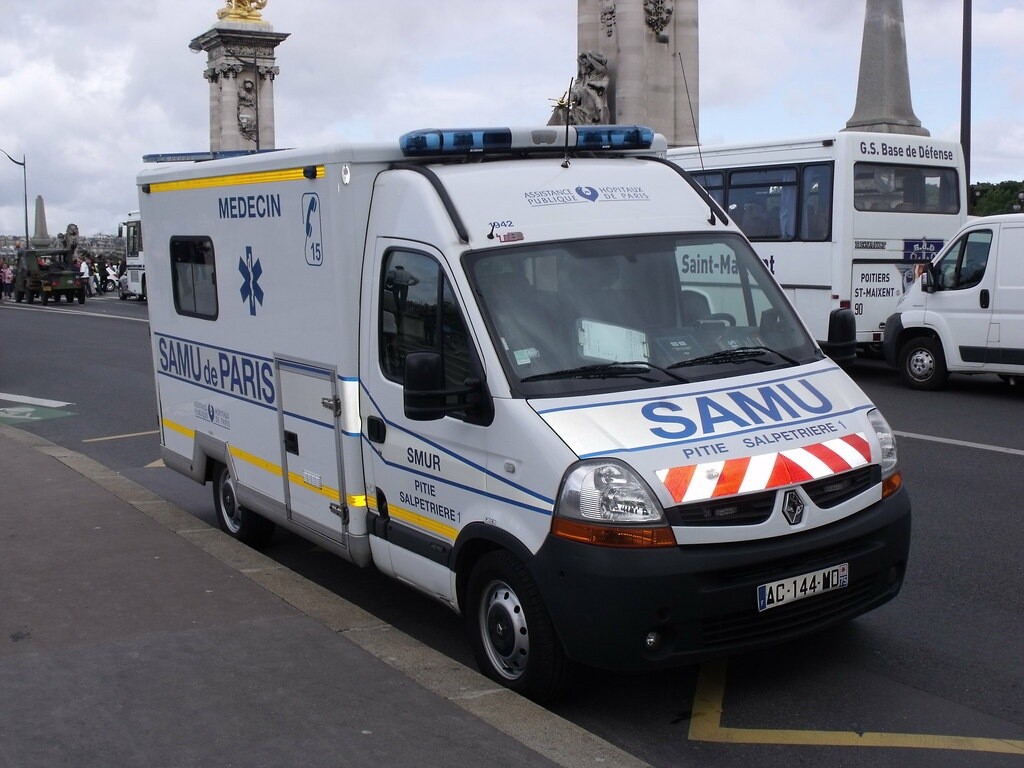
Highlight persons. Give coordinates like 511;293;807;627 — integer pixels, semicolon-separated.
382;264;437;376
0;256;19;303
73;250;127;299
559;51;612;127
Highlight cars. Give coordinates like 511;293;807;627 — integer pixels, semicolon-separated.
118;270;135;301
93;263;119;292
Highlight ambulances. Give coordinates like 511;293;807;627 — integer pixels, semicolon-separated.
136;53;913;691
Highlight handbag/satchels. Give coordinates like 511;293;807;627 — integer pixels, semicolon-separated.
99;270;110;277
385;271;397;291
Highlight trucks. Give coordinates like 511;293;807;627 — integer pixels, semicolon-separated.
883;213;1023;391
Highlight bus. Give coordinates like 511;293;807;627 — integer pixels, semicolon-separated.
668;132;967;357
118;210;147;301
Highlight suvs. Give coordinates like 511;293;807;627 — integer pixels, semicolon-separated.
14;248;85;305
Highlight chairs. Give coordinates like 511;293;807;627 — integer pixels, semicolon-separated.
487;272;546;343
571;259;647;317
735;201;831;239
855;200;958;215
